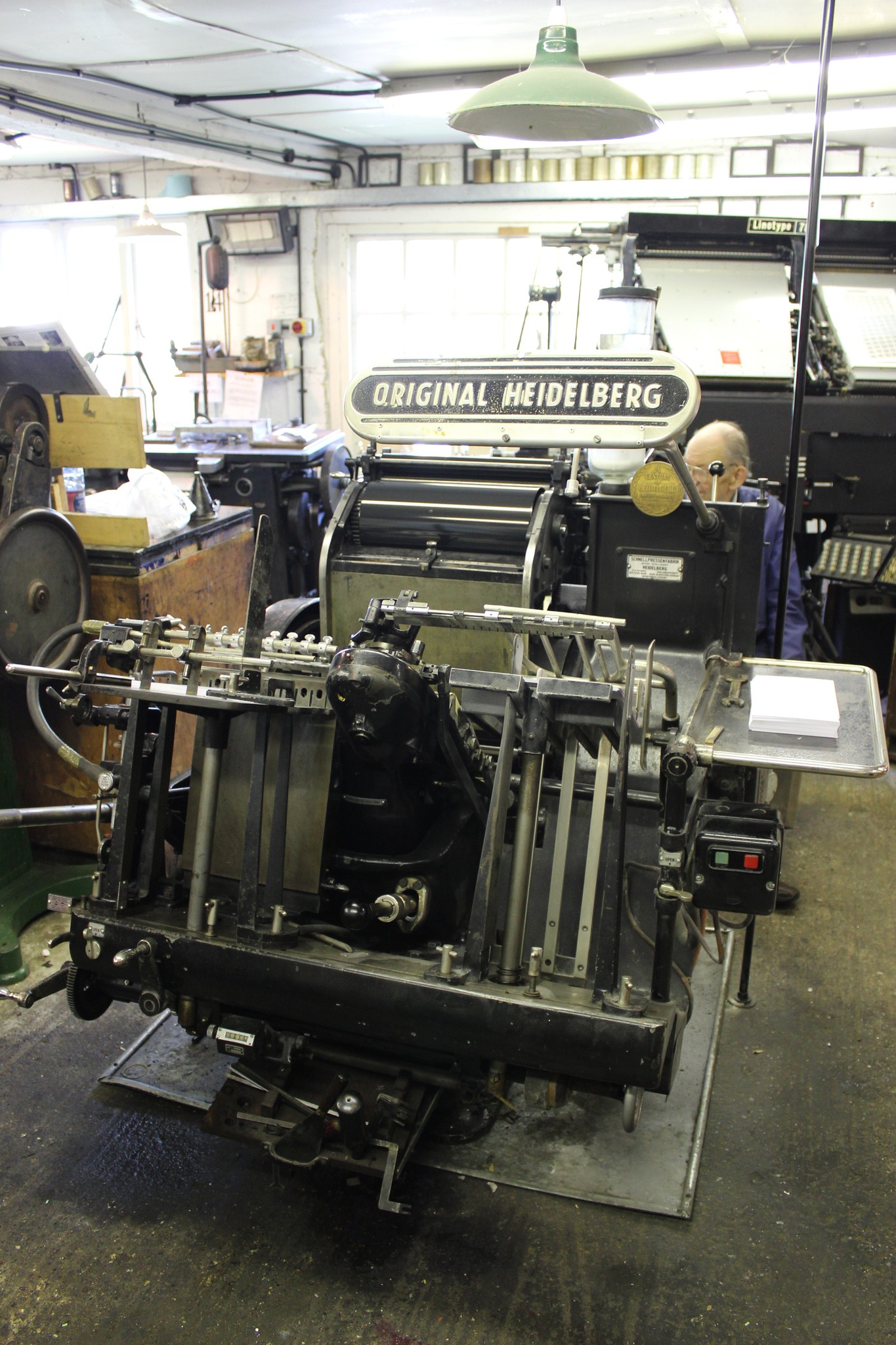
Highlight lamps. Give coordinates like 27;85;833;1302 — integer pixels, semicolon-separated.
119;156;181;239
445;1;665;143
206;206;295;257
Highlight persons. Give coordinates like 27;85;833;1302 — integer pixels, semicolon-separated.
683;421;808;661
